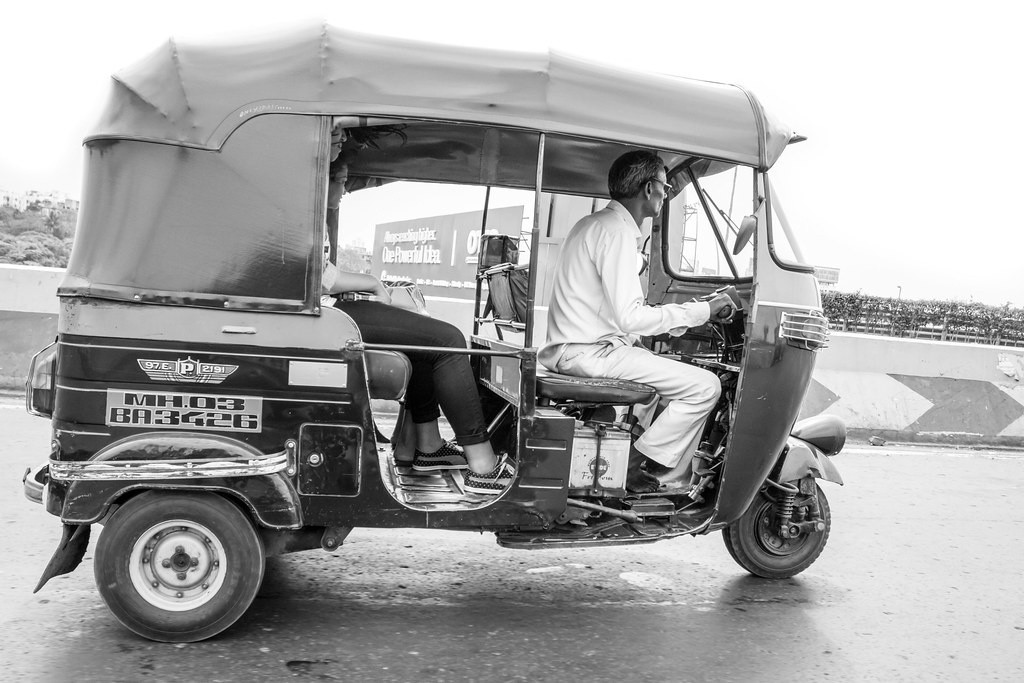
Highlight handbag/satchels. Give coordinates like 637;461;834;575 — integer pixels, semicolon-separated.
339;278;427;313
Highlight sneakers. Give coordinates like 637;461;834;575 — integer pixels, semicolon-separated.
413;437;469;471
464;453;518;495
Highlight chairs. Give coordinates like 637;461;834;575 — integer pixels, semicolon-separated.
485;262;654;411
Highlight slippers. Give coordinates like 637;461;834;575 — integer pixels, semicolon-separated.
394;459;413;467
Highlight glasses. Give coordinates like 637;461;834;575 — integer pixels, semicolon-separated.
641;176;674;194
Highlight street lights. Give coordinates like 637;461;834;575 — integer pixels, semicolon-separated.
897;285;902;298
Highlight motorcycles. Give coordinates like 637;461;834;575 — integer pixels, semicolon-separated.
21;21;853;646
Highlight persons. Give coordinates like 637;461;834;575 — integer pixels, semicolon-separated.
537;148;738;494
322;124;516;495
